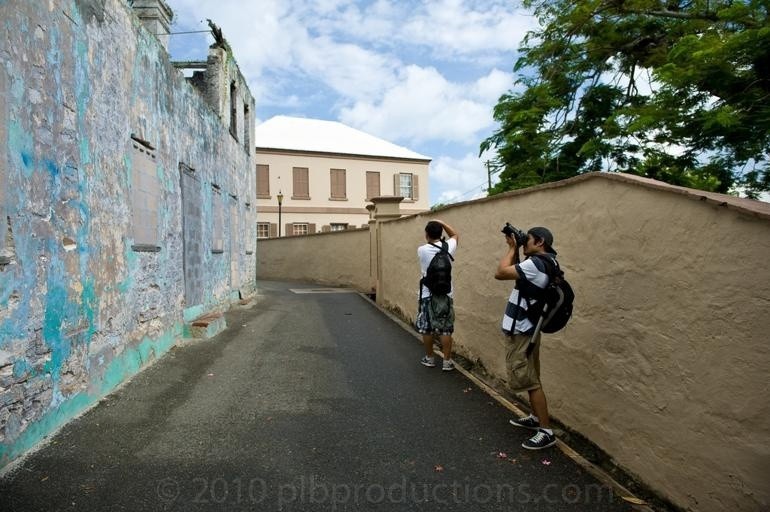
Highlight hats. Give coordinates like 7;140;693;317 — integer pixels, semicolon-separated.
528;226;557;255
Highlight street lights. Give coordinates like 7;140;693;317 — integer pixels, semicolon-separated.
277;190;284;237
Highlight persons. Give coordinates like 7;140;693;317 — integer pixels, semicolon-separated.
494;226;560;452
417;218;459;371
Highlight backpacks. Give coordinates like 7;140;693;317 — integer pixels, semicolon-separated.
419;240;451;312
527;255;575;334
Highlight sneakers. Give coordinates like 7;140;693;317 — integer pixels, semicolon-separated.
521;428;556;449
420;355;436;367
510;416;539;430
441;358;455;371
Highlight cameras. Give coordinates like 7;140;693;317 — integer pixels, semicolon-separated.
501;222;529;248
